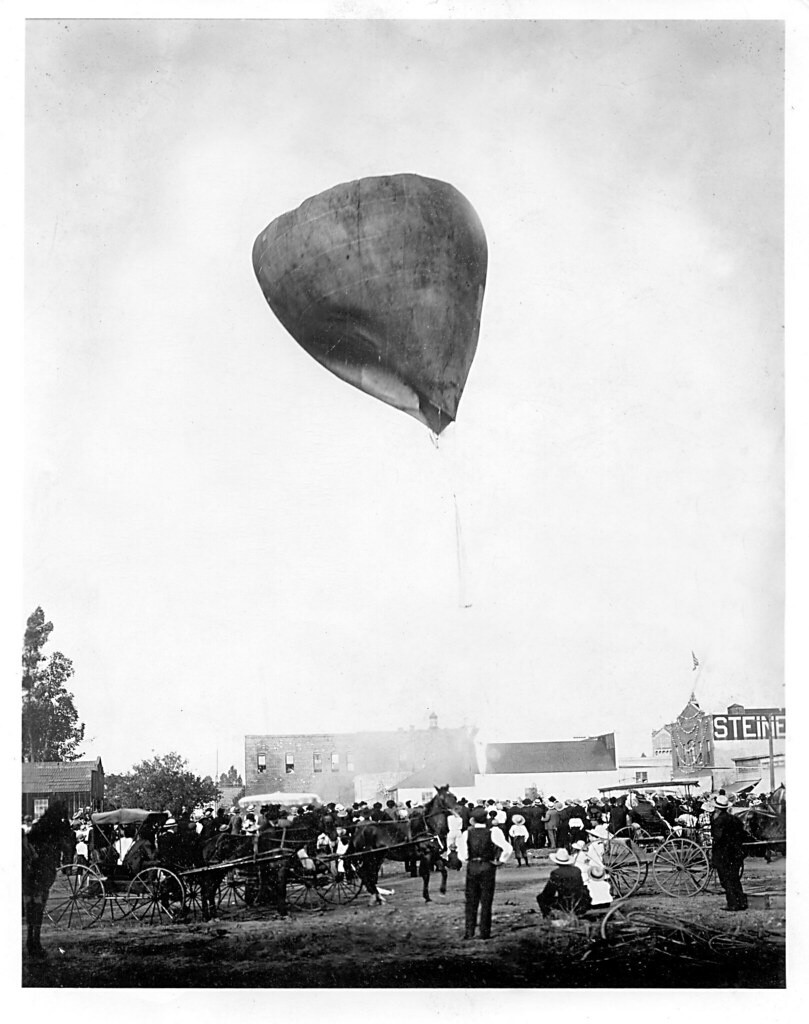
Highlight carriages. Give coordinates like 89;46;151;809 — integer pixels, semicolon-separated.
582;780;787;898
208;784;472;903
42;807;340;928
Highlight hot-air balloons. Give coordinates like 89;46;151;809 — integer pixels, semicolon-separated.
251;175;488;436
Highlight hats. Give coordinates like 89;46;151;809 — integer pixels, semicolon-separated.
587;867;606;881
711;796;732;808
549;848;574;864
23;801;427;842
449;796;714;826
586;829;603;838
572;840;587;850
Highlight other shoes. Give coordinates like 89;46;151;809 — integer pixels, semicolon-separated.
720;904;750;911
462;933;491;941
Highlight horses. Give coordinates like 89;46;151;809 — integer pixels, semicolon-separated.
353;783;457;906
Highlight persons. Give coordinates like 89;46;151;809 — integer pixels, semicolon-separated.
537;848;592;926
22;790;786;894
582;866;613;911
456;808;513;940
710;795;749;910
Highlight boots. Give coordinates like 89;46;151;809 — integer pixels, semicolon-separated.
523;857;530;867
517;858;522;867
